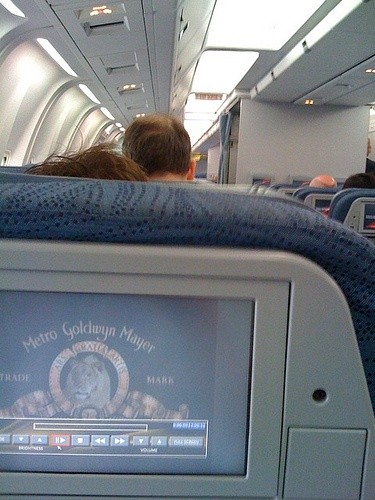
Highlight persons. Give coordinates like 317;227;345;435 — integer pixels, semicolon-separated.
123;114;196;181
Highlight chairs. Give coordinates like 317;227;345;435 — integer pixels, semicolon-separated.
0;165;375;500
